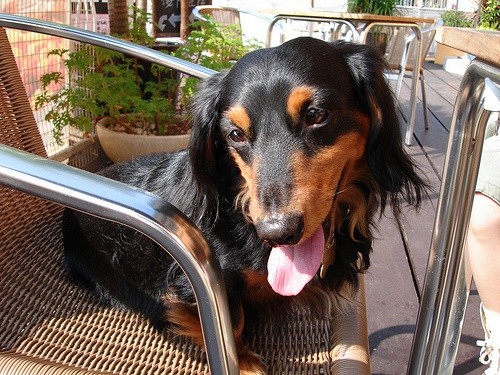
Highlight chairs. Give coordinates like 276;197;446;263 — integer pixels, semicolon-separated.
181;5;284;113
0;14;370;375
381;29;436;129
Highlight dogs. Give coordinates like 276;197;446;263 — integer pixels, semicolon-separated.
58;33;424;375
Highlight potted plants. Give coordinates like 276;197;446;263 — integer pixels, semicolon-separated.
346;0;409;56
33;4;267;165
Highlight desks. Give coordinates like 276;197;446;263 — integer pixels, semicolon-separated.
404;27;500;375
265;13;435;146
